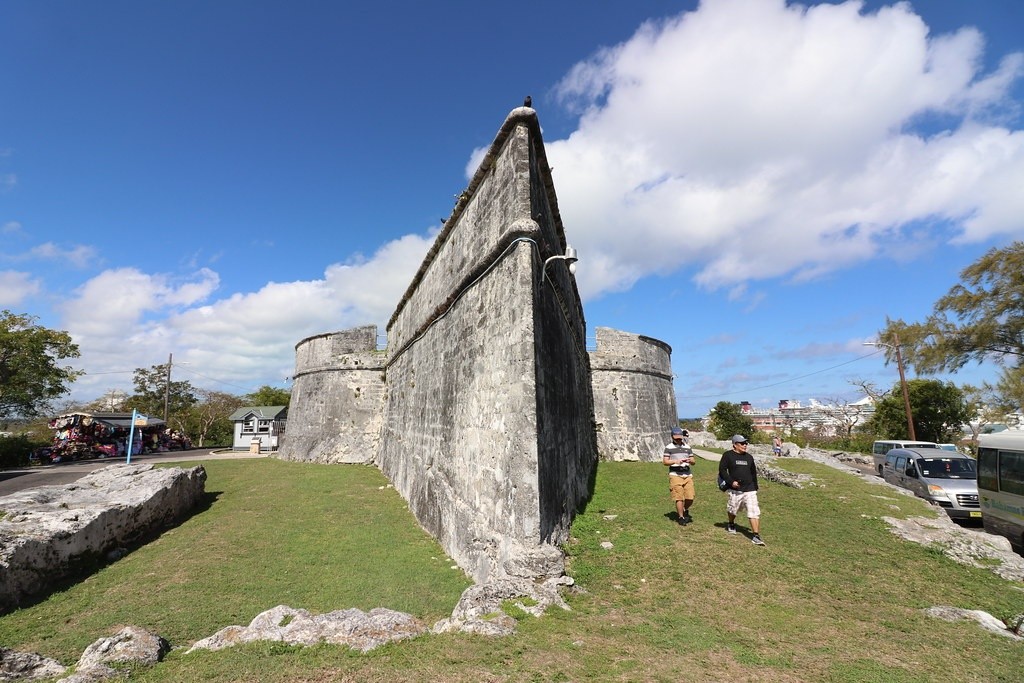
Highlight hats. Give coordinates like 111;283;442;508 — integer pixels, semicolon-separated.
732;435;749;444
671;427;684;440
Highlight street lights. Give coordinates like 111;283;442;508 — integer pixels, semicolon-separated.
862;342;916;442
164;360;193;421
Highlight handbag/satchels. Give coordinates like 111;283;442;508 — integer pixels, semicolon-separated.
717;472;729;492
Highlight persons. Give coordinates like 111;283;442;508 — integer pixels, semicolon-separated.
150;432;161;447
718;435;766;546
126;432;134;456
772;436;781;457
664;426;696;526
136;428;145;455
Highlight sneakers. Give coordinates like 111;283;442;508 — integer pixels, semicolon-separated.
750;534;766;546
727;522;736;534
678;516;686;526
683;507;688;517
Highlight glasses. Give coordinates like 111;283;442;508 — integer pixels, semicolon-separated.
736;442;748;446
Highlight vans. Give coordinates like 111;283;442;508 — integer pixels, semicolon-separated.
883;448;982;519
873;439;942;476
939;444;958;451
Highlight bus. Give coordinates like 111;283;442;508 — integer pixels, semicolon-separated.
975;428;1024;559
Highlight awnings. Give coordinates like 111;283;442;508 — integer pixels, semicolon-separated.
102;418;167;428
